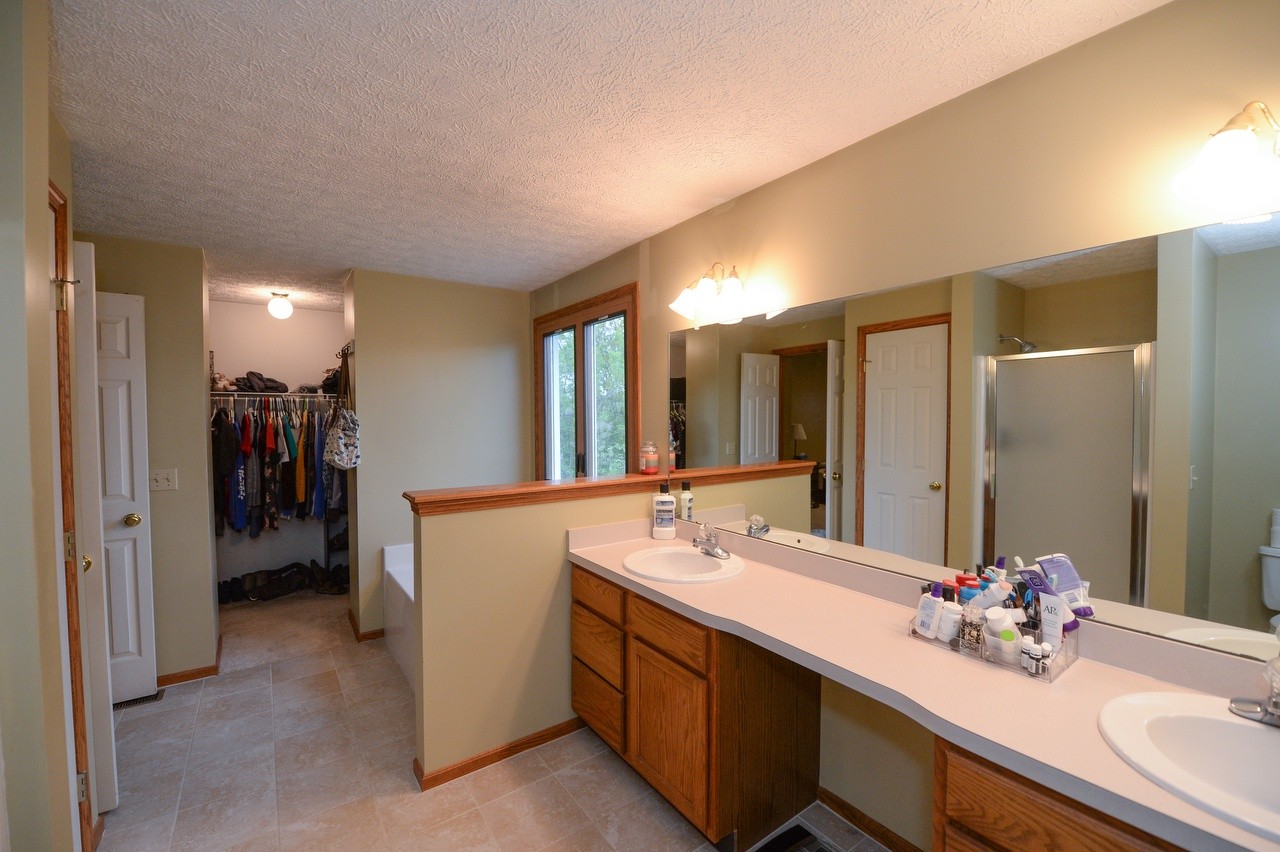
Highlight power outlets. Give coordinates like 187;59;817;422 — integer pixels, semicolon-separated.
150;468;177;491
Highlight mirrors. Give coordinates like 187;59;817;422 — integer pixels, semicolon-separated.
668;210;1280;662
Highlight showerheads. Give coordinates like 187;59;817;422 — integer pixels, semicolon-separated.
999;334;1035;353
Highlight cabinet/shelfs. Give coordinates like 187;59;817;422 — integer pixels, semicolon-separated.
932;734;1190;852
569;561;821;852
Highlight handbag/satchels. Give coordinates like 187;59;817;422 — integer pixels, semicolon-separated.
323;408;361;470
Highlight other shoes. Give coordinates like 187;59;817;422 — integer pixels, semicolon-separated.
311;560;328;583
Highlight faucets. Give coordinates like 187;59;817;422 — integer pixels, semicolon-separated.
747;514;770;539
1228;625;1280;726
692;522;731;559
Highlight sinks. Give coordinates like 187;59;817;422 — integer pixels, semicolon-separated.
623;545;745;584
1099;693;1280;843
740;529;829;554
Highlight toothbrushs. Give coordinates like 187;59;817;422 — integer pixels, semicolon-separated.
1014;555;1024;569
984;569;1016;602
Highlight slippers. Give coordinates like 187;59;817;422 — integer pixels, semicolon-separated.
316;580;347;595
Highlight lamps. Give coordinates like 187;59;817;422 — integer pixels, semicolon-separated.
999;334;1036;353
668;262;787;330
792;423;807;460
1211;102;1280;138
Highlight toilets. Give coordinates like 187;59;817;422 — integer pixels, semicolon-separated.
1258;544;1280;634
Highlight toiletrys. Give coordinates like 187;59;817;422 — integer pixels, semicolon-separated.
1038;591;1066;658
917;582;945;639
1035;552;1094;619
680;480;694;521
652;483;677;540
994;555;1008;578
985;606;1023;641
1015;567;1081;633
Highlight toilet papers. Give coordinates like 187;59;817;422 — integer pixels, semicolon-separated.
1269;508;1280;548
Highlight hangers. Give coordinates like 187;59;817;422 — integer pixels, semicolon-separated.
210;396;320;430
